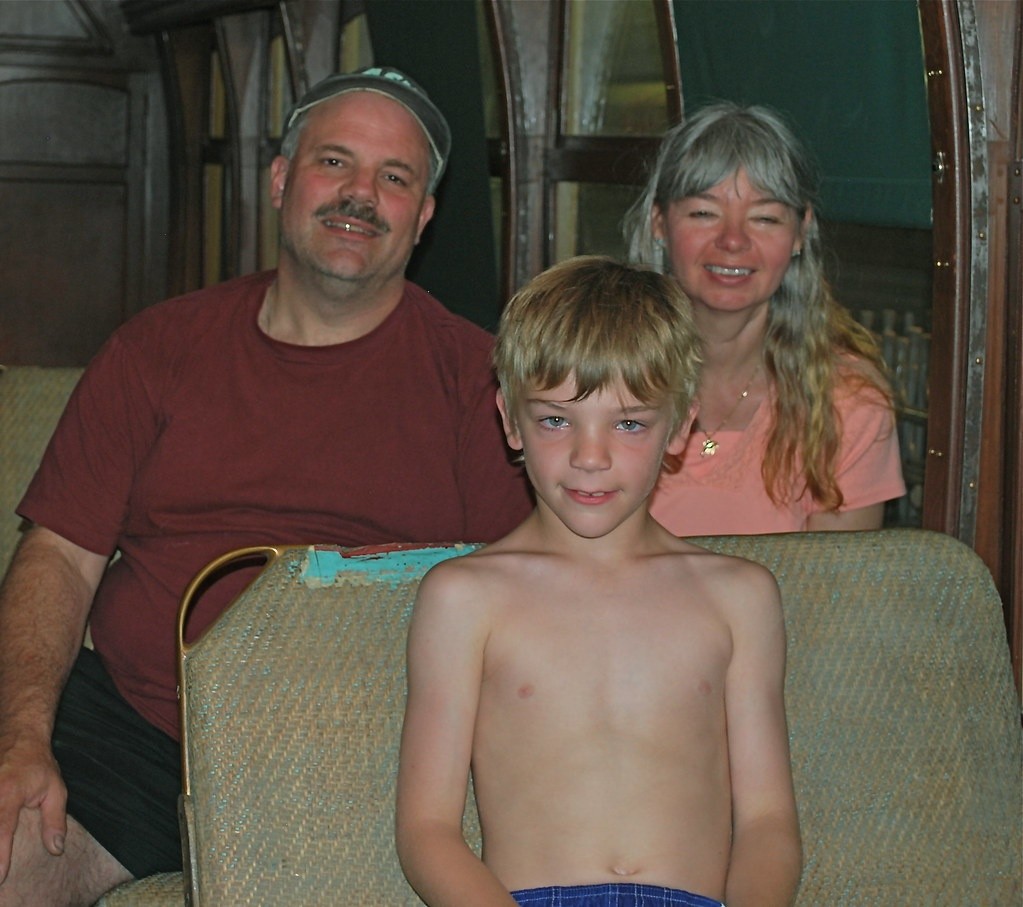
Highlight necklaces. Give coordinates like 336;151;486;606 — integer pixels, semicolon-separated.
695;358;758;457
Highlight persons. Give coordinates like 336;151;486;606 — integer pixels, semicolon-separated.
396;255;804;907
1;69;537;902
625;100;907;537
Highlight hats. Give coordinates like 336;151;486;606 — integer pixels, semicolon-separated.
286;64;453;191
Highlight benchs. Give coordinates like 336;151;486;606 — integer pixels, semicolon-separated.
174;527;1023;907
